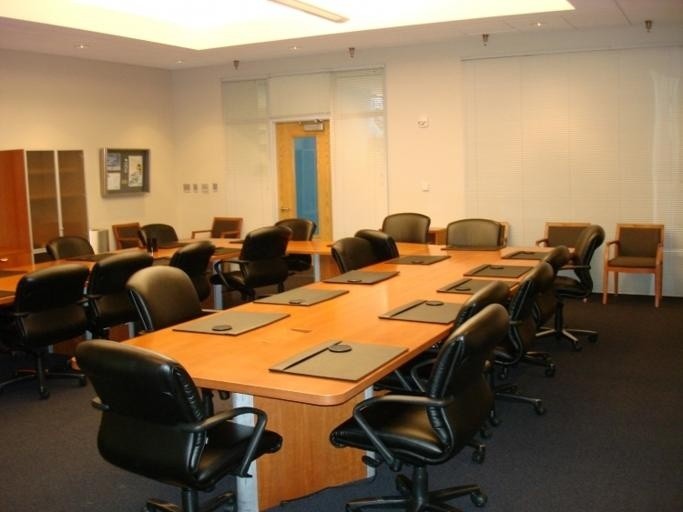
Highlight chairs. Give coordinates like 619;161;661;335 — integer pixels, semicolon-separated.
125;264;223;335
0;265;100;400
328;303;510;511
75;339;283;512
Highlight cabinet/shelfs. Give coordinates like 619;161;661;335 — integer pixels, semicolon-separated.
1;150;90;265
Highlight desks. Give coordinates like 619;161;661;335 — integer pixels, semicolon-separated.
0;239;575;512
